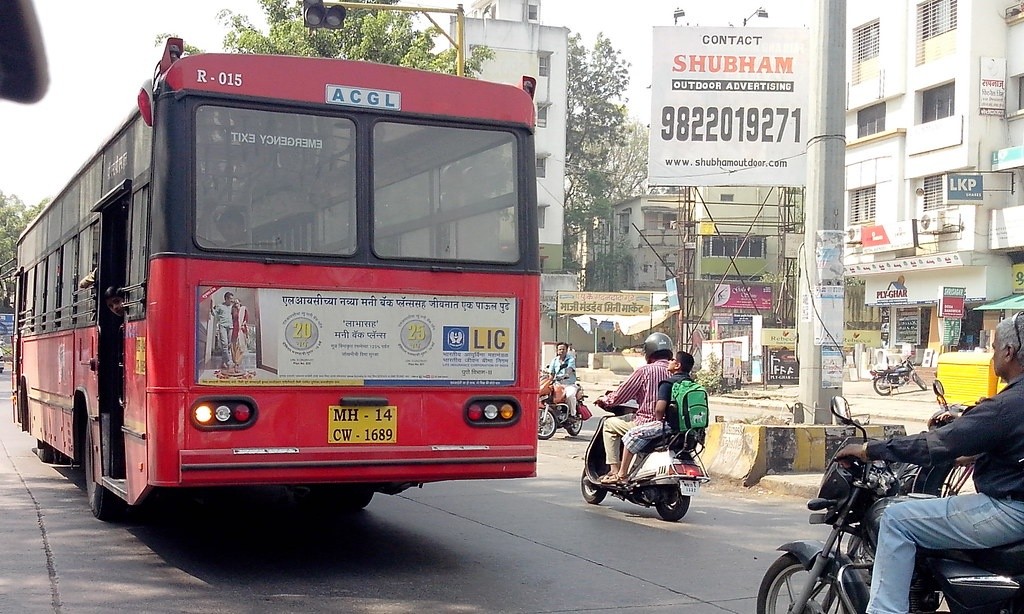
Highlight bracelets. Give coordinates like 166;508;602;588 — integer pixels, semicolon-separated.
863;442;868;458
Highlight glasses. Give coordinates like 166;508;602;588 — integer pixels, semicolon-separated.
1012;311;1024;354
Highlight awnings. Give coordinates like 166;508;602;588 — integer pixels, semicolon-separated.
972;294;1024;310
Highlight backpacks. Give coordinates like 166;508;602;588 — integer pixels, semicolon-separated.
667;377;708;432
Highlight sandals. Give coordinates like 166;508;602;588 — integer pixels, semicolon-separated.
597;473;615;481
601;475;626;484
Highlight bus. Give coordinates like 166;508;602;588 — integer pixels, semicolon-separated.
0;36;542;522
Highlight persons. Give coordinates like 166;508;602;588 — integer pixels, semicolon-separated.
607;350;697;485
78;266;98;289
598;336;608;352
607;342;614;352
567;342;577;358
836;313;1024;614
594;333;691;483
549;340;581;421
210;292;251;371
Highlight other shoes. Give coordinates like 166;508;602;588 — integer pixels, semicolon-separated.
568;415;575;422
222;361;235;369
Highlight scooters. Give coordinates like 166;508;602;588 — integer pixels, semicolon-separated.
580;380;710;522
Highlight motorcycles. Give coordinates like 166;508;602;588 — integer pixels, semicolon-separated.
537;363;588;440
869;353;927;396
756;374;1024;614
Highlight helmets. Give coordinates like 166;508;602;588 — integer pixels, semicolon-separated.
644;333;673;363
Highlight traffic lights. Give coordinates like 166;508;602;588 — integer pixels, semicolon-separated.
303;0;347;29
